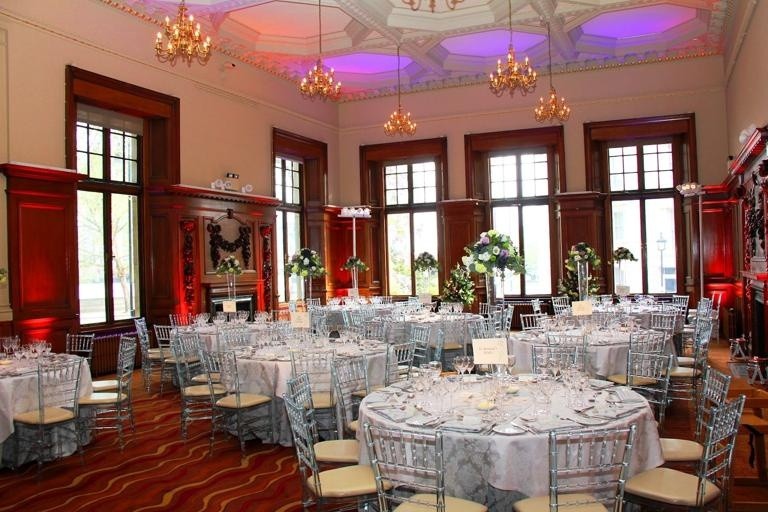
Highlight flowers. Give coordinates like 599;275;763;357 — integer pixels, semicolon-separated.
214;254;244;276
286;248;327;277
563;241;601;270
462;228;525;273
339;255;371;271
406;250;436;274
607;246;638;264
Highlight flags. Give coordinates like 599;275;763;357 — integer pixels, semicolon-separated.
484;271;506;304
615;261;630;295
350;267;360;289
576;261;590;295
226;272;237;293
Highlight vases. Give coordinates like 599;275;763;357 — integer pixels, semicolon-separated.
339;255;371;271
484;271;506;304
576;261;590;295
350;267;360;289
462;228;525;273
607;246;638;264
563;241;601;270
615;261;630;295
226;272;237;293
214;254;244;276
286;248;327;277
406;250;436;274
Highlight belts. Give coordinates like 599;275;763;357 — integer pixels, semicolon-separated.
365;357;591;426
0;334;51;370
190;295;463;354
542;292;662;337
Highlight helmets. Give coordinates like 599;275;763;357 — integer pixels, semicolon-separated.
493;380;649;436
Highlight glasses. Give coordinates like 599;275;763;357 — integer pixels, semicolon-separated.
532;21;571;124
382;45;419;140
737;124;757;144
300;2;343;105
150;0;212;65
486;0;540;98
675;181;705;302
210;173;253;194
336;206;373;257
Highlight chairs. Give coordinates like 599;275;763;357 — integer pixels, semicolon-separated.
0;291;766;512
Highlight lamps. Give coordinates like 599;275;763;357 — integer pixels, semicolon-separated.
210;173;253;194
150;0;212;65
382;45;419;140
532;21;571;124
300;2;343;105
336;206;373;257
486;0;540;98
737;124;757;144
675;181;705;302
